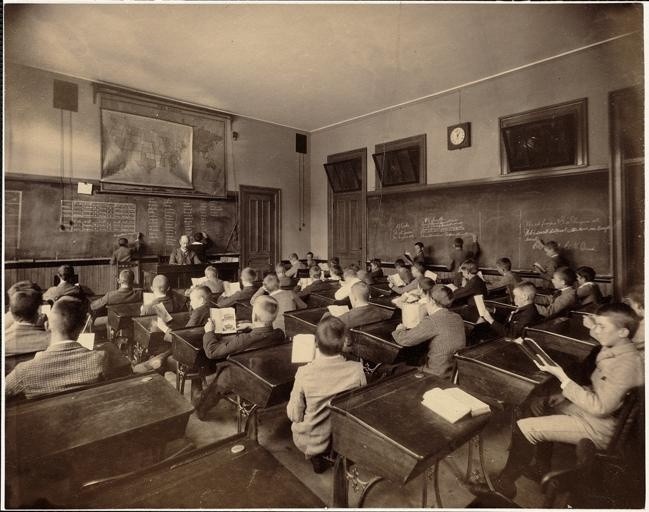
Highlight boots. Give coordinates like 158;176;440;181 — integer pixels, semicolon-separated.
524;403;553;482
191;365;226;418
133;348;172;373
471;423;536;500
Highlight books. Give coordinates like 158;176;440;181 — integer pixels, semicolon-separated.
513;337;558;369
151;302;173;323
386;273;406;287
209;306;238;334
291;333;318;364
473;294;488;317
222;280;241;296
400;302;428;329
403;252;414;263
327;304;350;318
421;387;491;424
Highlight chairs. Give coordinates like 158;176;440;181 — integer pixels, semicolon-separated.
549;386;645;508
461;435;602;509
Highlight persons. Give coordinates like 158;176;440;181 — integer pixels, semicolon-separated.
575;265;603;306
275;262;292;287
191;294;280;419
468;300;644;500
306;251;317;268
184;266;224;297
5;295;114;399
91;269;142;329
352;264;374;285
327;266;344;280
391;284;466;375
189;232;212;262
217;267;259;308
396;277;435;322
582;282;645;350
388;262;426;295
533;266;576;317
5;289;51;357
43;264;84;300
168;234;201;265
320;281;384;348
140;274;174;316
5;278;42;328
250;273;308;333
297;266;322;291
133;286;213;374
109;231;141;278
394;258;412;284
285;315;367;473
334;269;361;301
453;257;487;304
286;252;306;278
534;237;562;289
493;258;521;294
369;259;384;278
447;234;479;271
481;281;540;338
411;241;426;268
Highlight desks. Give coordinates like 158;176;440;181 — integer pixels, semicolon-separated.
326;366;504;507
446;303;507;347
226;341;310;443
233;297;259;323
571;301;613;317
5;371;197;486
366;294;406;323
349;316;429;380
47;431;333;511
524;315;605;387
485;284;509;301
85;293;109;335
166;286;202;314
104;302;145;346
281;306;343;347
204;291;227;311
137;261;240;294
451;333;579;416
310;287;355;311
3;341;133;388
369;281;396;298
530;285;563;306
130;311;196;368
169;320;255;395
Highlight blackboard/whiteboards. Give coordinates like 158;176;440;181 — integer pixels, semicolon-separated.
5;172;241;269
365;168;613;285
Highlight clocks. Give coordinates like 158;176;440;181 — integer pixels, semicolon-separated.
445;121;473;151
